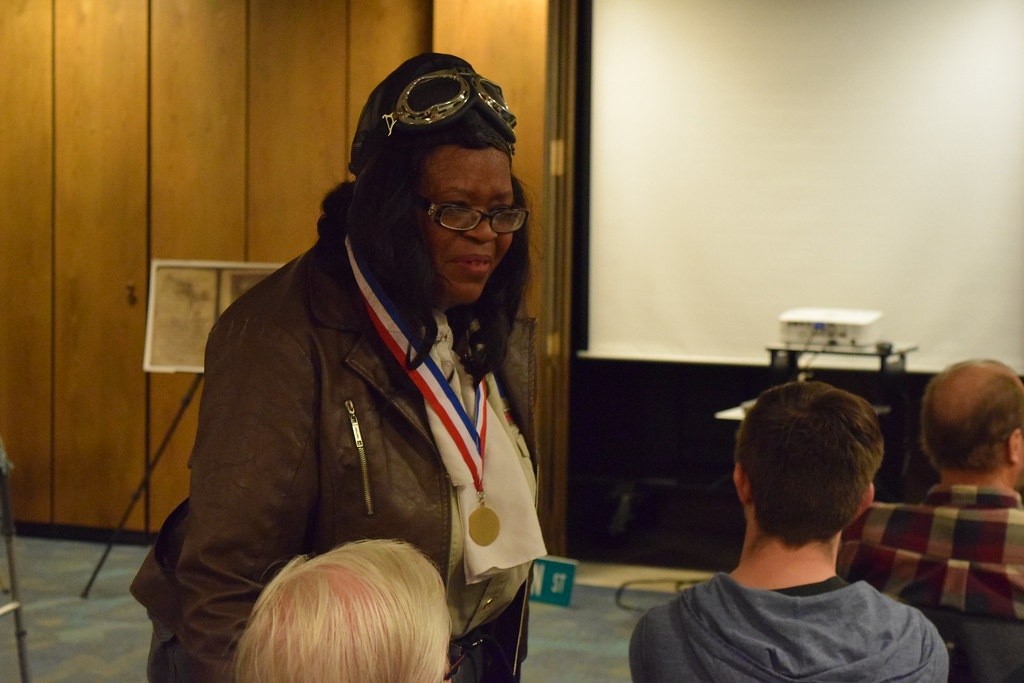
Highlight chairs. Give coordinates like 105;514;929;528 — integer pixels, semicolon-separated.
922;607;1024;683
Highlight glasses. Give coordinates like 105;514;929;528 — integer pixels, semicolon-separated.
412;193;525;234
381;66;522;145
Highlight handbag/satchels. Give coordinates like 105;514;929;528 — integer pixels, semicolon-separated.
132;496;189;683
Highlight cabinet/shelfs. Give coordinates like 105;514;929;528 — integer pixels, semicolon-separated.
715;343;918;499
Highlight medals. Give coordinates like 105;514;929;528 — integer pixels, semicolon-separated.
469;506;501;546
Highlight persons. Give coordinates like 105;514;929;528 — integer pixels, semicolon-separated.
628;380;950;682
838;359;1023;624
131;52;549;683
236;539;451;683
